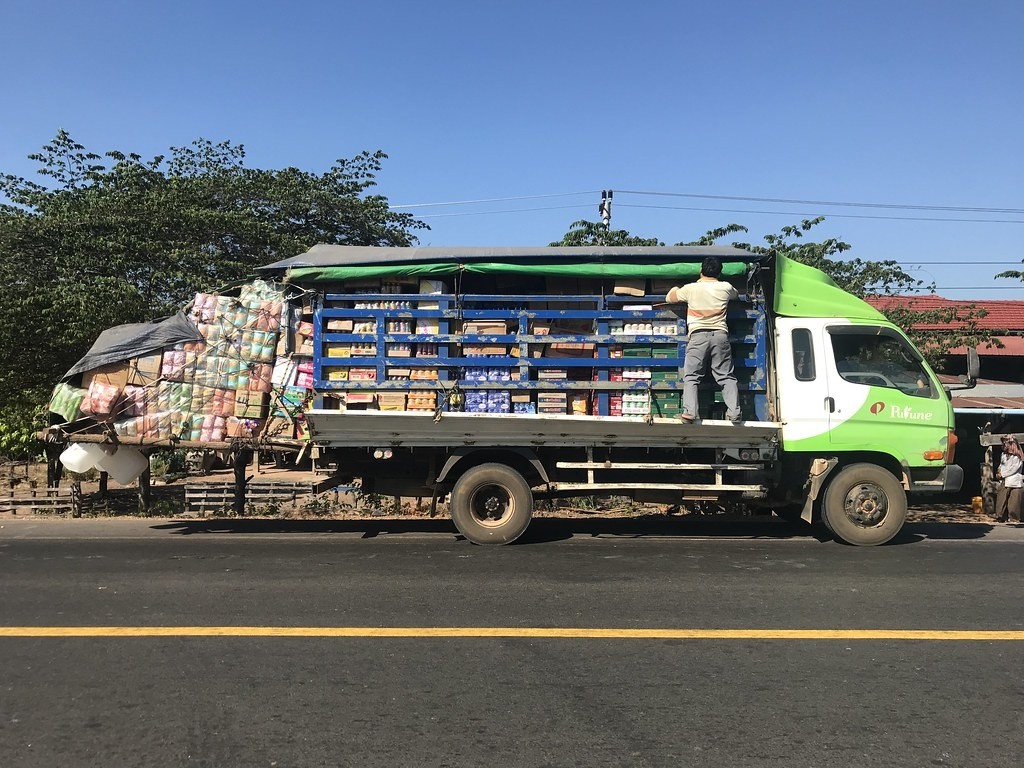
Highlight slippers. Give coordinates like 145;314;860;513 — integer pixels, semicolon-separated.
673;413;695;424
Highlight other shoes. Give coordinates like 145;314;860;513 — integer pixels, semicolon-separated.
1005;519;1021;524
989;513;1003;522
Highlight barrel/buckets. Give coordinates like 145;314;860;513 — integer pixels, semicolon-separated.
972;497;983;514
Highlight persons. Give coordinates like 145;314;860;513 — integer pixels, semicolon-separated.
993;434;1023;525
666;256;741;423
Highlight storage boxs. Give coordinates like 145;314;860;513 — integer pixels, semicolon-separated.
127;349;162;387
297;373;313;388
297;419;310;439
266;418;294;439
289;334;304;354
234;390;264;418
297;359;314;373
271;356;298;386
226;417;262;438
301;345;314;355
324;274;724;417
82;361;129;391
270;384;306;418
299;321;314;336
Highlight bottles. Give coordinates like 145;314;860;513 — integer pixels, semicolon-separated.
60;444;149;484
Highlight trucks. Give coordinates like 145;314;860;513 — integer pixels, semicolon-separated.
300;248;981;548
